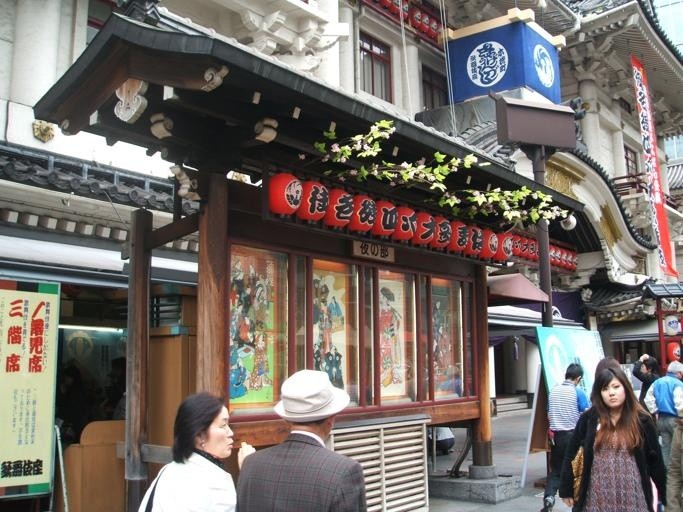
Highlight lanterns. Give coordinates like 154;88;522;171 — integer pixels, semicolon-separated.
494;233;578;269
432;217;498;258
269;170;354;228
666;341;680;363
348;195;434;244
375;0;445;41
664;315;680;336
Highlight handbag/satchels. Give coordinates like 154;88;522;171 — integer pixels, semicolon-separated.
644;383;657;414
568;445;586;501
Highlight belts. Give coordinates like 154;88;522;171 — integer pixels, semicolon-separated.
657;411;672;416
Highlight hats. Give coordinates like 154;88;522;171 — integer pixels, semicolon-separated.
667;360;683;372
270;369;351;421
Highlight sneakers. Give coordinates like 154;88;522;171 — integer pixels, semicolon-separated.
540;496;555;512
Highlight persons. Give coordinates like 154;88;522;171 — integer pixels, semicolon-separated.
236;366;368;512
378;285;404;387
136;389;258;512
423;298;454;386
540;352;681;511
228;257;272;393
312;277;345;389
